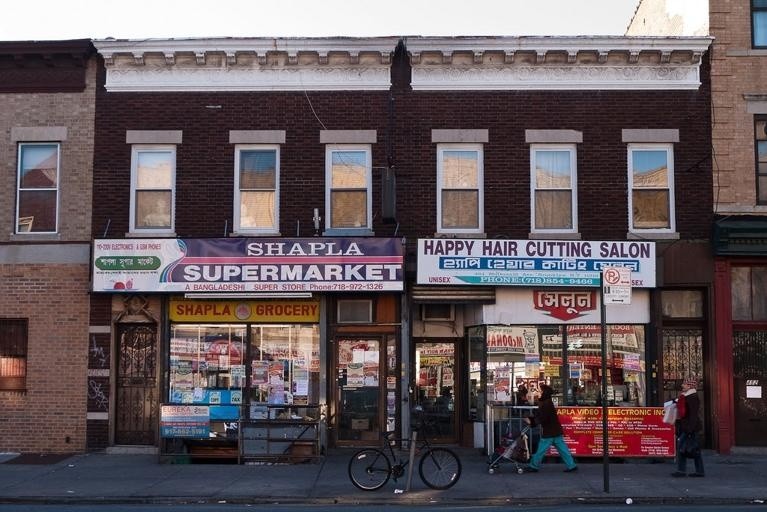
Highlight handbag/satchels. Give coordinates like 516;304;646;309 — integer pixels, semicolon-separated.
663;404;677;425
510;447;528;462
677;433;703;458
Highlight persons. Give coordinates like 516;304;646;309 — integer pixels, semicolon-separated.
521;384;580;474
670;374;704;479
514;385;537;413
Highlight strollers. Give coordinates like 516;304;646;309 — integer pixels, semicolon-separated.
482;421;533;474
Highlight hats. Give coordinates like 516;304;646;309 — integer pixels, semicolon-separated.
682;377;698;386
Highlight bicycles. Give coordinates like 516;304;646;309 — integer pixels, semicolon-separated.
347;418;461;491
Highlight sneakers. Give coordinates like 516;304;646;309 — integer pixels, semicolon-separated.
523;467;539;473
671;473;685;478
688;473;705;476
564;465;578;473
